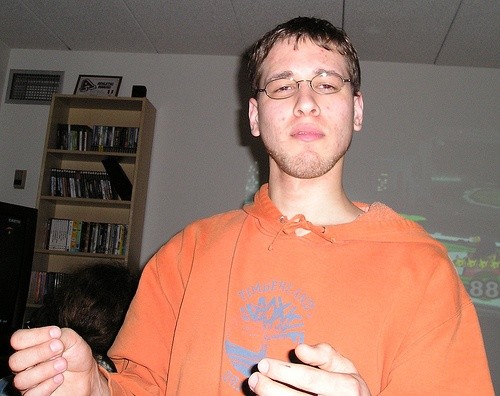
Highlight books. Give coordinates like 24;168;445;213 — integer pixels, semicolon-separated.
43;217;129;255
55;122;140;153
34;271;71;304
50;157;132;201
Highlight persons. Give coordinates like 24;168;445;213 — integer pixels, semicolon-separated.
0;261;139;395
6;16;496;395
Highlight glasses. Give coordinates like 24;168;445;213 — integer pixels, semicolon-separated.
254;72;356;99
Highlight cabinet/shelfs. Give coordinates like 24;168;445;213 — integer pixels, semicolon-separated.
24;91;160;327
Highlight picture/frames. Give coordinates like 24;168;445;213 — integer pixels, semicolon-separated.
72;74;124;96
